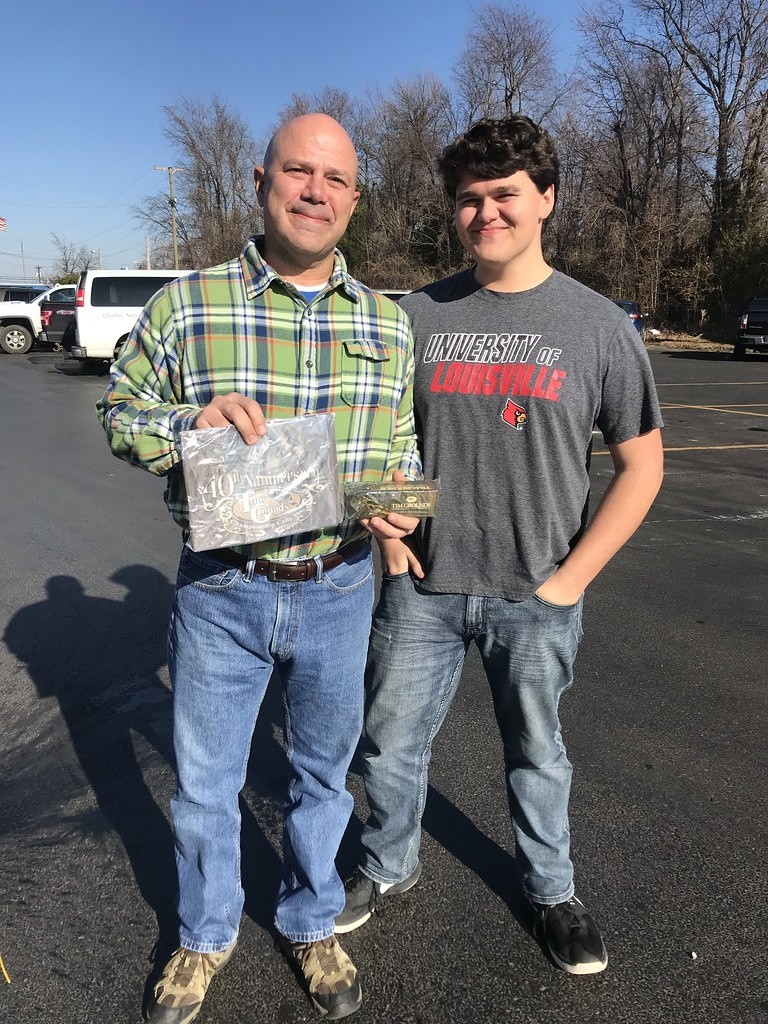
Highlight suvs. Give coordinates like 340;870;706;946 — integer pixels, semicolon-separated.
0;285;74;303
0;283;79;354
735;296;768;361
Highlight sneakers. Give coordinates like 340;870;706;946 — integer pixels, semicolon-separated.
529;894;608;975
332;864;420;934
278;932;363;1020
145;936;238;1024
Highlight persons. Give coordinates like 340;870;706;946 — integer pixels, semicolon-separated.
326;115;665;973
95;113;426;1024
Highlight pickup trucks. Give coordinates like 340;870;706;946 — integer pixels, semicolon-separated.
38;300;74;353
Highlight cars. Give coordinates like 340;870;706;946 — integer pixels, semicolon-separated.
372;288;413;303
616;299;649;345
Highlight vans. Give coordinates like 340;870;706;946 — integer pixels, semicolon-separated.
71;269;197;360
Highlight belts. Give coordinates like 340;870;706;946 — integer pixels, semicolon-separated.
213;536;371;582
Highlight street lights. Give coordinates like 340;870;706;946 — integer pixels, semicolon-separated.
91;249;103;270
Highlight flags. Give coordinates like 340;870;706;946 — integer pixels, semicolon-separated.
0;217;7;232
